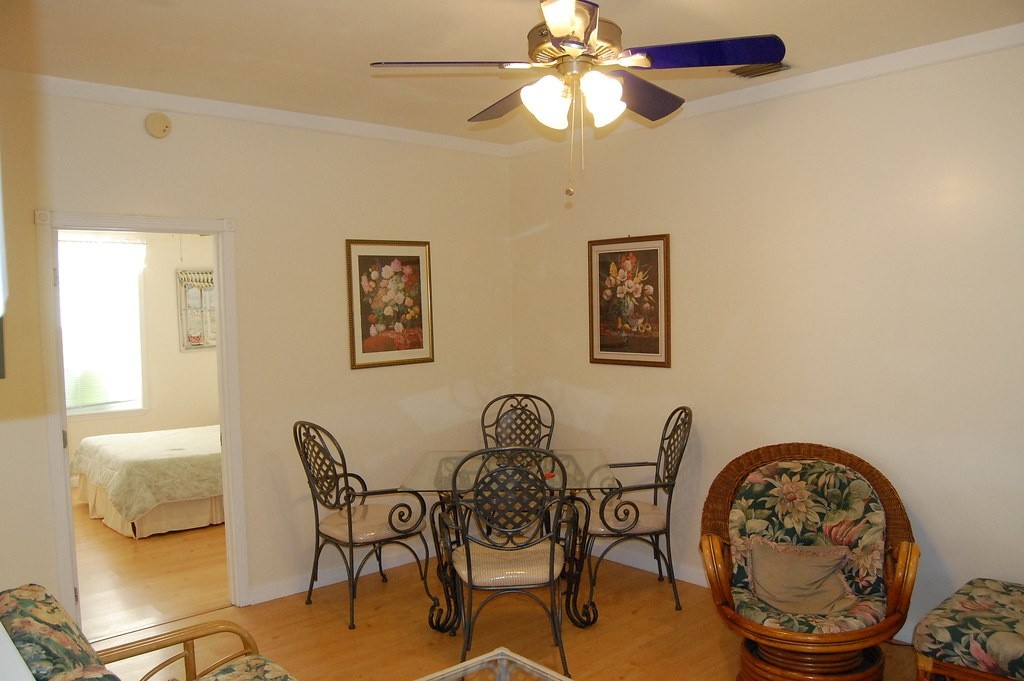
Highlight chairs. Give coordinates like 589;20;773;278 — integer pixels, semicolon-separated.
293;420;439;629
577;404;694;616
0;581;299;681
437;446;591;678
481;393;555;543
697;442;922;681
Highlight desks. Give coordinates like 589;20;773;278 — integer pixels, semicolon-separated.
414;646;575;681
396;449;618;497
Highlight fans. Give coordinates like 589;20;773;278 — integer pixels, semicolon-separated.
369;0;787;123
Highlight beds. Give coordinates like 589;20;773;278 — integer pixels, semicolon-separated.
69;425;225;540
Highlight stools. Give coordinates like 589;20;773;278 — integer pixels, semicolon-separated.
911;577;1024;681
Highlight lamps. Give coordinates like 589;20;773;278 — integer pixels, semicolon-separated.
522;20;626;195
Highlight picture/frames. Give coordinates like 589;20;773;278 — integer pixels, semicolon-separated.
345;238;434;370
588;233;672;369
